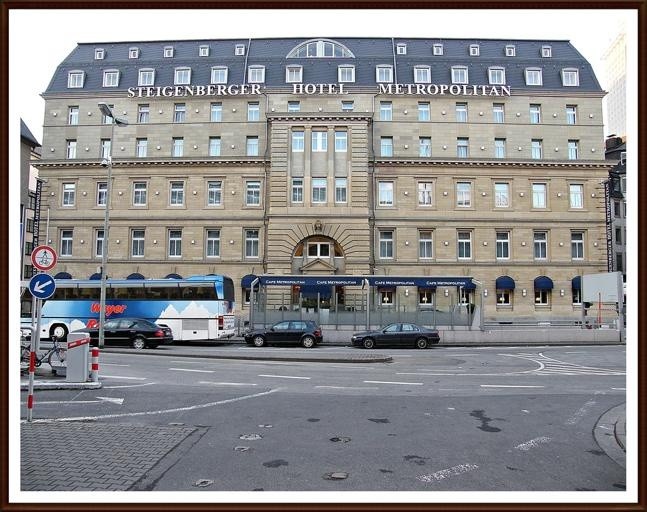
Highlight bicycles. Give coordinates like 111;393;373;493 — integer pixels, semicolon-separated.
21;335;66;374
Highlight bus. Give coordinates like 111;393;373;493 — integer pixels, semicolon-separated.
21;275;237;341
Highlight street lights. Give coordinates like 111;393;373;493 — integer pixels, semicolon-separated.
97;102;130;349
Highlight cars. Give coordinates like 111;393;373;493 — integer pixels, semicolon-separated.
71;318;174;348
245;320;323;348
352;323;440;349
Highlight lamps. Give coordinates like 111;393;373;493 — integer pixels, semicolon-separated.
315;220;322;231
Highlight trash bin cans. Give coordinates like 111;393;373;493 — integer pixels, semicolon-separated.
66;332;90;382
